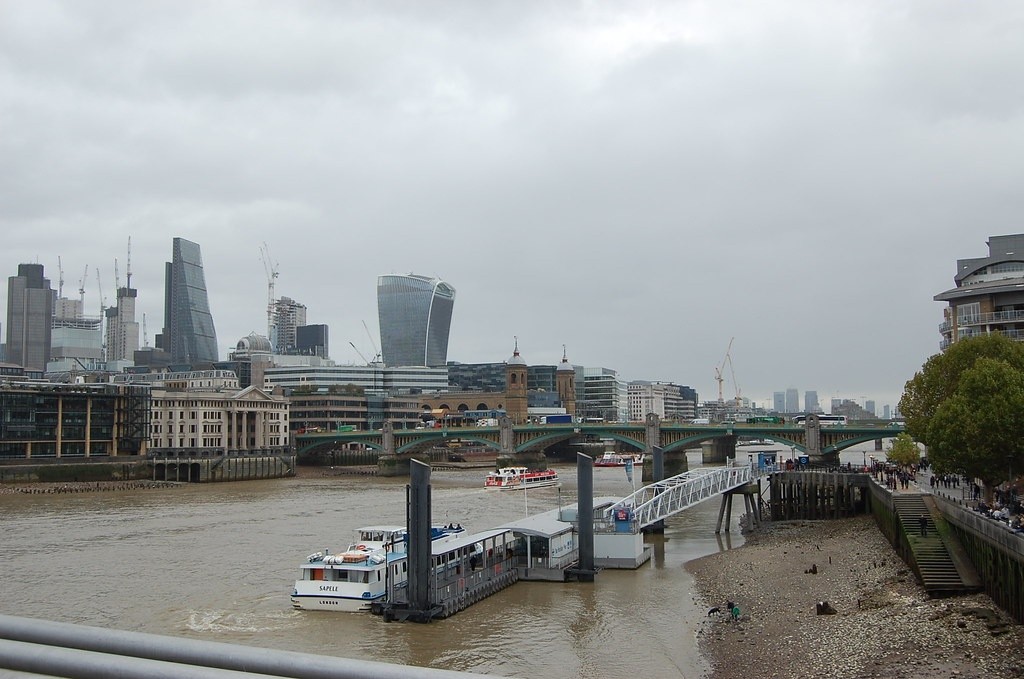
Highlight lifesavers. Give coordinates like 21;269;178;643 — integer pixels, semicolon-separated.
357;545;365;550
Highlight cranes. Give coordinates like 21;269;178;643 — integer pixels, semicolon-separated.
57;254;65;298
125;235;132;290
715;337;742;406
78;264;88;315
259;241;280;323
114;257;121;290
95;267;107;347
348;319;381;365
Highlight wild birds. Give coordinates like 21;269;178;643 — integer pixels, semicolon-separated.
707;607;721;616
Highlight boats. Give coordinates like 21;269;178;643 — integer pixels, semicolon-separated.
594;451;646;466
483;466;558;491
290;524;488;613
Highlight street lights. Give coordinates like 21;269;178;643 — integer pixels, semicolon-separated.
863;450;866;465
869;454;884;483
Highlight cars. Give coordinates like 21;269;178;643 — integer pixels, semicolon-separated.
720;421;734;424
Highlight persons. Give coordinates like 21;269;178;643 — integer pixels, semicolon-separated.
979;487;1024;531
973;483;980;500
786;458;800;471
470;555;477;571
885;467;909;490
930;473;957;489
919;515;927;536
449;523;462;530
733;607;739;622
726;600;734;617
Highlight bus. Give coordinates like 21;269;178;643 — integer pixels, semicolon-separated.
791;414;847;428
746;416;784;425
690;418;709;425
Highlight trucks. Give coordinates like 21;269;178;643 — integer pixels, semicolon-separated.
540;414;572;424
